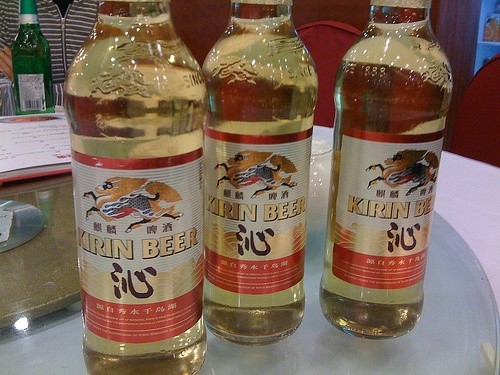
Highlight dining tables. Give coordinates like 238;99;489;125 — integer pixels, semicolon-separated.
0;104;500;375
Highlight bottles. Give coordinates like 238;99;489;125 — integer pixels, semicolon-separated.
319;0;453;340
62;0;208;374
11;0;54;115
200;0;320;345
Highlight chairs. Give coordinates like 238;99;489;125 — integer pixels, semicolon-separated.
291;20;363;128
448;52;500;168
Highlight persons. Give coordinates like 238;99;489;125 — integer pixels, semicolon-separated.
0;0;102;107
